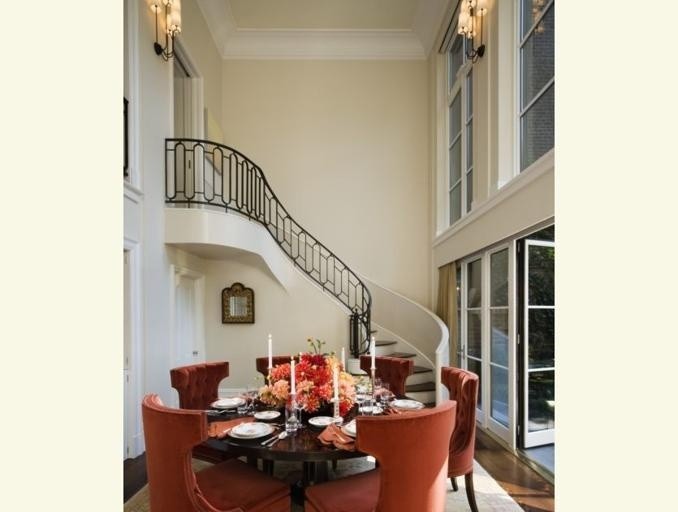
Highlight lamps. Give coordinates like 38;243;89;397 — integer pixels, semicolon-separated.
148;0;182;62
457;0;487;60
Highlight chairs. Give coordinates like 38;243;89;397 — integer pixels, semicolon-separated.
427;365;481;512
299;397;459;512
253;353;307;391
356;352;417;399
140;392;245;511
167;359;233;412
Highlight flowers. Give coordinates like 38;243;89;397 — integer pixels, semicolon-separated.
256;352;367;416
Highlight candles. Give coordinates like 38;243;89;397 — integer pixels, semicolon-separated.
288;355;297;393
331;363;340;398
267;335;273;369
367;337;378;368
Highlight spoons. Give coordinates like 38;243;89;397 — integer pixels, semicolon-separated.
266;429;289;447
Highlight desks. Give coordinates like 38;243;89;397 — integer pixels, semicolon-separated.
176;393;430;512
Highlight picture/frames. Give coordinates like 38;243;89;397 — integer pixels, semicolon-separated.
221;282;255;324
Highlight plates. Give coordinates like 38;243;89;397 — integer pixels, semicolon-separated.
340;419;357;438
226;421;276;442
210;397;247;410
359;405;384;415
388;398;427;412
306;415;337;428
253;410;282;421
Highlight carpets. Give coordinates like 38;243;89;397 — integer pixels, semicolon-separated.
124;454;525;512
